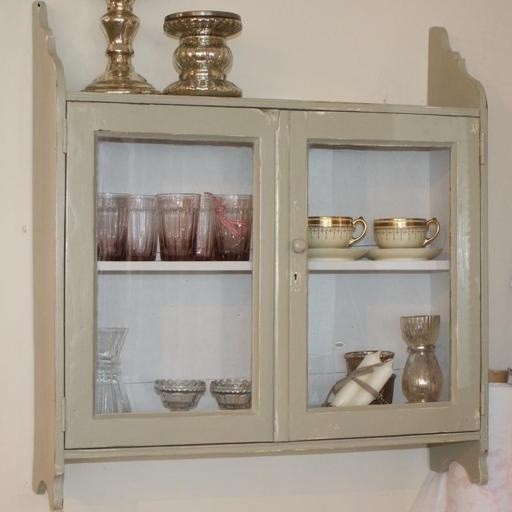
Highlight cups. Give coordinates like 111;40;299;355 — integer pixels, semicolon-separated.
157;193;213;260
345;350;396;405
126;194;160;262
374;217;440;249
307;215;367;249
211;193;253;262
94;192;126;261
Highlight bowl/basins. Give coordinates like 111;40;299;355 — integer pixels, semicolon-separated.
155;378;206;411
210;379;253;410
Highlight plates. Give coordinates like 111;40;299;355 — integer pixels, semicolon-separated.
366;245;444;262
306;247;369;262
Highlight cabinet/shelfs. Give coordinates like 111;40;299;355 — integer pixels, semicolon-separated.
57;94;484;463
